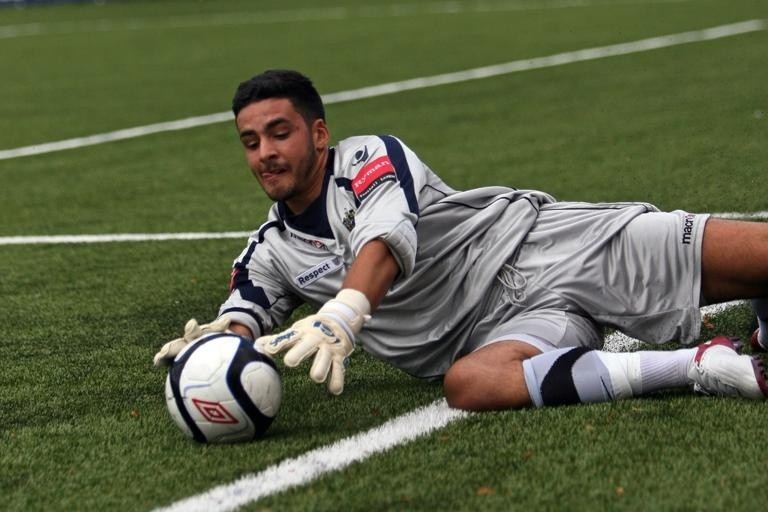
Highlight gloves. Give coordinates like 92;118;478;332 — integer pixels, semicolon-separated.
151;313;232;368
257;291;371;396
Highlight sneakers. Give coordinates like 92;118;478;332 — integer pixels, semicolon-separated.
690;327;767;402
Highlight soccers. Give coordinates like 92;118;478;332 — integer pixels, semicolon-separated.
165;332;283;445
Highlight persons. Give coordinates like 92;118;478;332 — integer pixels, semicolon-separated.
147;67;768;414
